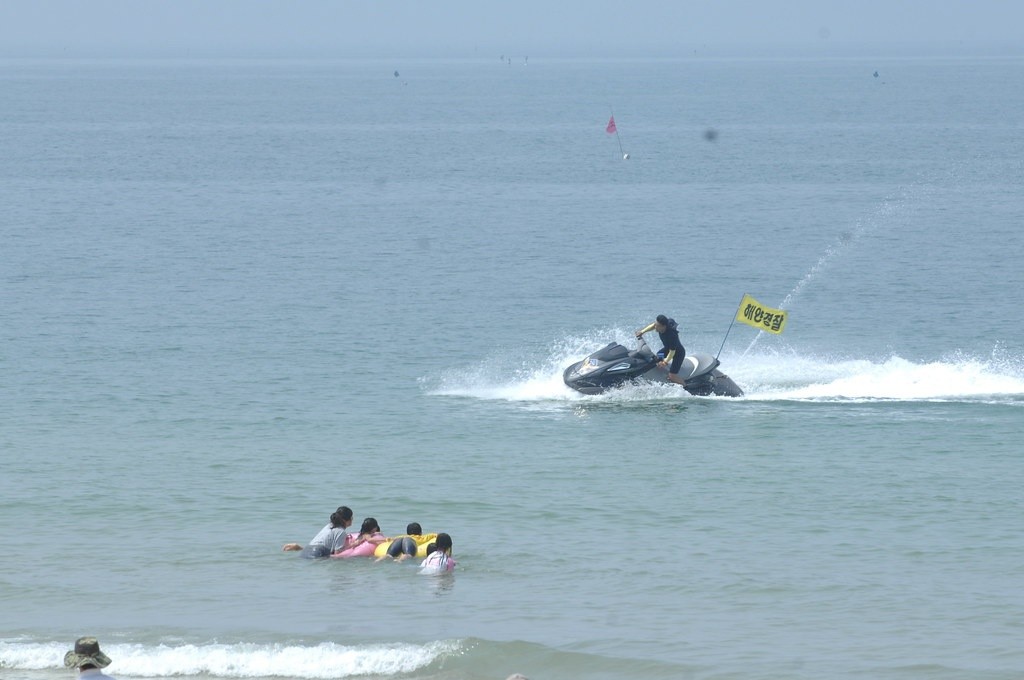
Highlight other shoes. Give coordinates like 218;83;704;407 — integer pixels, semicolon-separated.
683;386;689;391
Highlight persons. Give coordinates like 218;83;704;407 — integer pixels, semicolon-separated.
283;506;387;559
64;637;117;680
372;522;455;574
635;314;686;388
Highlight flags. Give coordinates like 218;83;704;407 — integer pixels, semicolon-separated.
607;117;617;132
736;295;786;335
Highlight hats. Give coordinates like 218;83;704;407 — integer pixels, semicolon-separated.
64;636;112;670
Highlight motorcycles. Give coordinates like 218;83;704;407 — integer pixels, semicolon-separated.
563;332;744;397
